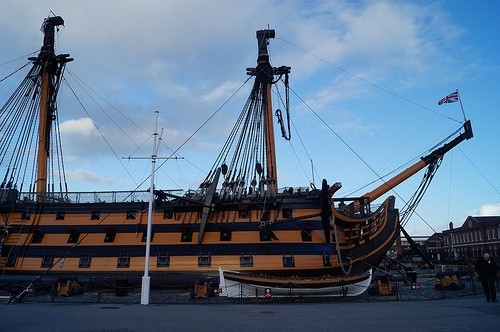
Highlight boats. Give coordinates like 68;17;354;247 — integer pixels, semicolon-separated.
213;264;373;300
0;9;474;300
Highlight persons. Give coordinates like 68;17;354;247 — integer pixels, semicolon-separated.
477;253;498;302
